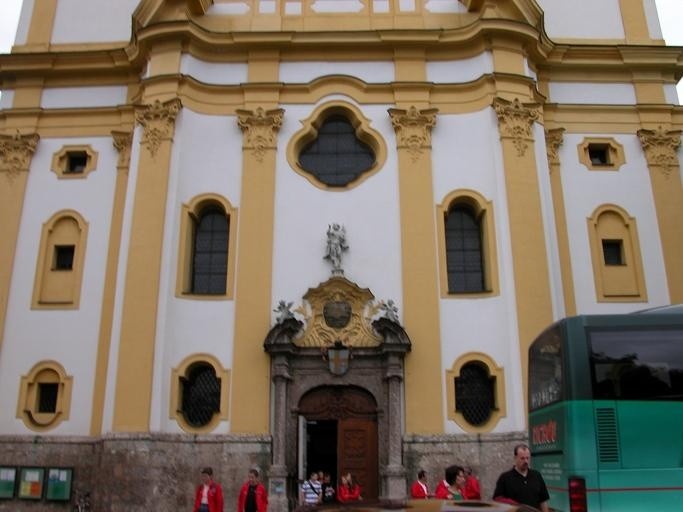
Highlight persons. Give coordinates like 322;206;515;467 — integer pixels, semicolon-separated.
435;464;466;500
462;466;481;501
237;469;268;512
492;445;549;512
300;471;363;504
192;466;224;512
410;470;435;498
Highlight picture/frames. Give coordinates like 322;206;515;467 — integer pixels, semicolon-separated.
16;465;46;501
44;466;74;502
0;465;18;500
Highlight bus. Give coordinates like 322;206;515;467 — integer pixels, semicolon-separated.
528;303;683;512
